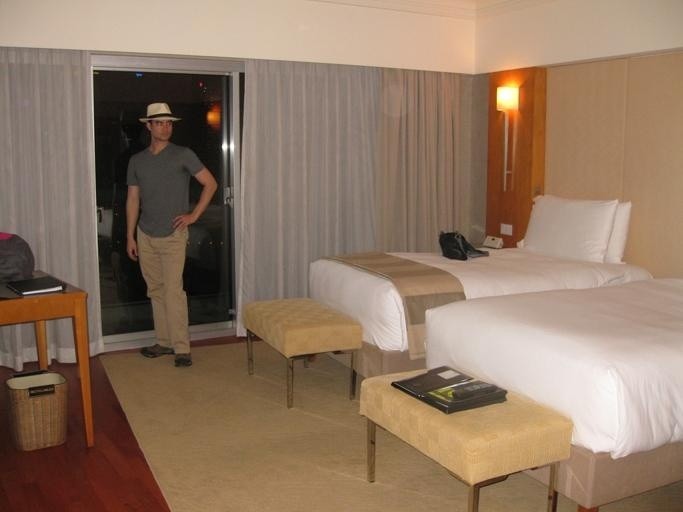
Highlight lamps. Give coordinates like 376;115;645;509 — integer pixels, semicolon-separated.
493;83;519;196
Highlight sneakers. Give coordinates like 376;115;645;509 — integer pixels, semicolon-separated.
139;344;175;359
174;352;193;366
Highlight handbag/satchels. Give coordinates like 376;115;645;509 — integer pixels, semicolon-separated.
437;231;490;260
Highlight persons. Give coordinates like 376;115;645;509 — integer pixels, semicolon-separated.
125;102;217;367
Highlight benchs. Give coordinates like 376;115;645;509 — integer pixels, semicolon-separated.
232;296;364;410
356;367;576;511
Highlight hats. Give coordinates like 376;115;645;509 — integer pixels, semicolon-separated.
138;102;182;123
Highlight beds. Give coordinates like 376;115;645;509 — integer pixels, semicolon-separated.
423;273;682;511
303;197;655;380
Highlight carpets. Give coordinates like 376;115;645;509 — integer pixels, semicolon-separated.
96;334;683;512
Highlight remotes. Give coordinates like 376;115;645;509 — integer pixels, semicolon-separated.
452;383;497;399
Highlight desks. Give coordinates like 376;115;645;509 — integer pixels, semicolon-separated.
0;268;96;452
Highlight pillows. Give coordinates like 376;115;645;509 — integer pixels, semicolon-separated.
520;193;617;262
516;201;635;264
